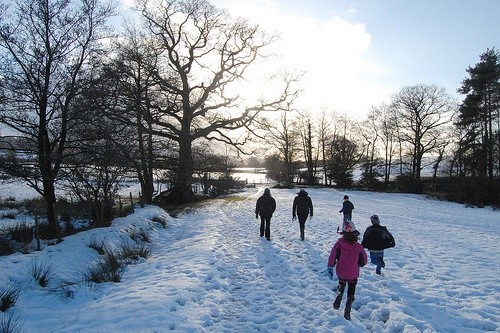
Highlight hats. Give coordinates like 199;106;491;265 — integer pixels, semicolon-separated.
370;214;380;224
300;189;305;192
342;221;356;232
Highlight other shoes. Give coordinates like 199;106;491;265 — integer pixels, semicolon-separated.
344;308;350;320
376;271;381;274
333;292;343;309
379;260;385;267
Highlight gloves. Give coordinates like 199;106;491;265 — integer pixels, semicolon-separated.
326;268;334;279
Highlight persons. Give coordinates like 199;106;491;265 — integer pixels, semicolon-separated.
292;188;314;241
255;188;276;242
339;195;354;227
327;219;368;320
361;214;395;275
